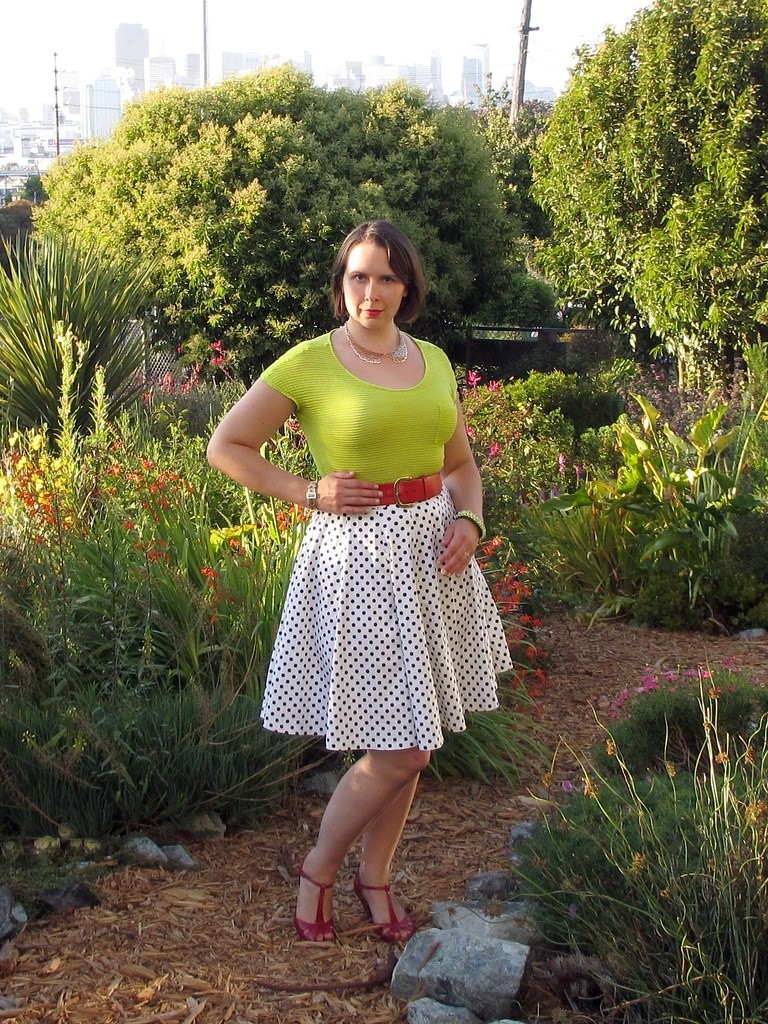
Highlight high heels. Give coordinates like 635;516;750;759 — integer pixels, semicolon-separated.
295;861;337;943
354;867;413;942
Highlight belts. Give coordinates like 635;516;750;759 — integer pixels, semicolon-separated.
378;472;443;509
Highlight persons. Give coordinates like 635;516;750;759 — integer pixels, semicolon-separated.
204;220;513;941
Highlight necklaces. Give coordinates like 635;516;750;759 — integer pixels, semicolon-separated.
343;321;408;365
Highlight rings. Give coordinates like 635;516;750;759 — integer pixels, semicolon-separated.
464;552;469;558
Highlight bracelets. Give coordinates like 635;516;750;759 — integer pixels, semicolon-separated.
306;481;318;509
455;510;486;542
